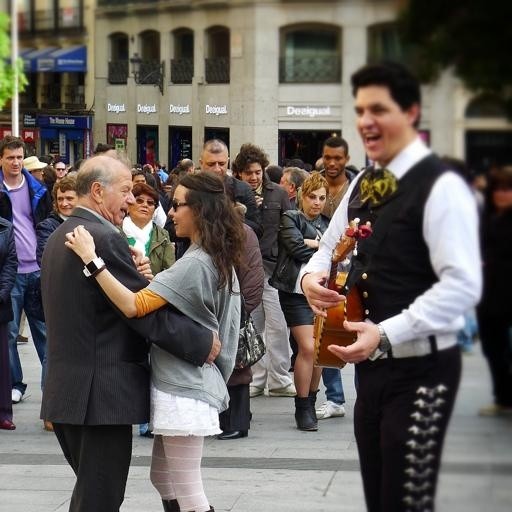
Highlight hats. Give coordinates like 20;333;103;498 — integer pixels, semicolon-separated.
24;156;47;171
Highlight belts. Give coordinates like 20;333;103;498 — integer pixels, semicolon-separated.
367;330;456;361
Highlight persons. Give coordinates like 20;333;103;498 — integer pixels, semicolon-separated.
116;183;176;438
1;136;78;434
95;144;201;228
217;222;264;440
176;139;261;262
265;158;324;184
63;172;248;512
40;155;222;511
299;61;483;512
440;155;512;418
280;167;310;372
231;142;297;398
316;137;360;419
268;172;339;431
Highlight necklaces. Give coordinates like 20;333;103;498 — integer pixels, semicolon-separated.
328;183;345;204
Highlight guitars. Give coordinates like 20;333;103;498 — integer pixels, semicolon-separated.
312;217;373;370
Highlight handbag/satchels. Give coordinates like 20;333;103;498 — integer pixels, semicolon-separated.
234;297;266;371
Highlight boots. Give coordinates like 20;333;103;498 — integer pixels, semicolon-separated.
294;391;318;431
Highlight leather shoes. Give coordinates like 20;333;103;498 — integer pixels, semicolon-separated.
0;420;16;429
218;430;249;440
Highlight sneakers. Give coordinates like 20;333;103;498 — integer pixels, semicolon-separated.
479;406;512;417
11;389;24;404
315;400;346;419
17;334;28;342
269;383;297;398
250;387;264;398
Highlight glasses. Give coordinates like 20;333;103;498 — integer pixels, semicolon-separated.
136;198;155;206
172;199;186;212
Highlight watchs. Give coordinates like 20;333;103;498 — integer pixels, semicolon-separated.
376;324;391;352
83;258;105;277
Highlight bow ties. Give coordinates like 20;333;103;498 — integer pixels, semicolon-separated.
349;168;394;209
